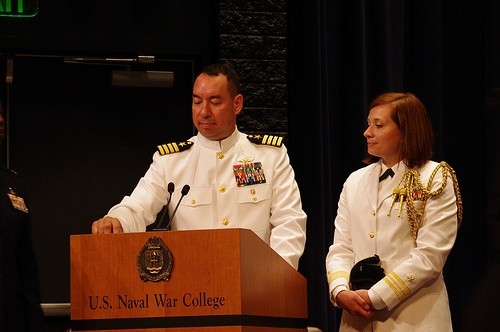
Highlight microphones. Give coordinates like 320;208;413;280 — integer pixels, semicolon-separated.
155;182;190;230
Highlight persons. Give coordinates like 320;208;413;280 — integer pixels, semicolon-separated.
325;93;463;332
0;113;48;332
91;62;306;271
